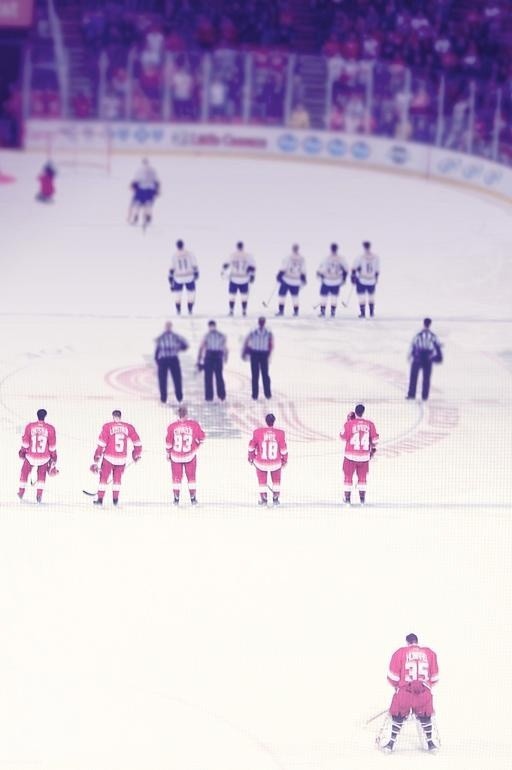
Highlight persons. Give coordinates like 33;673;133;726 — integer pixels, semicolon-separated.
153;238;385;405
125;157;164;234
246;415;290;506
2;2;511;165
403;318;444;402
32;162;57;204
166;409;204;504
339;404;378;506
382;635;440;751
90;410;143;504
16;410;58;503
